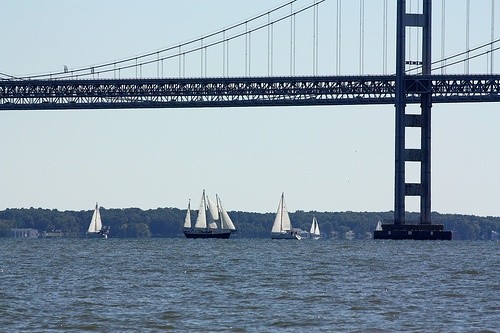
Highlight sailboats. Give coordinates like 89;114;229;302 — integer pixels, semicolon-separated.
181;188;237;240
306;217;320;241
269;192;300;241
86;202;108;238
